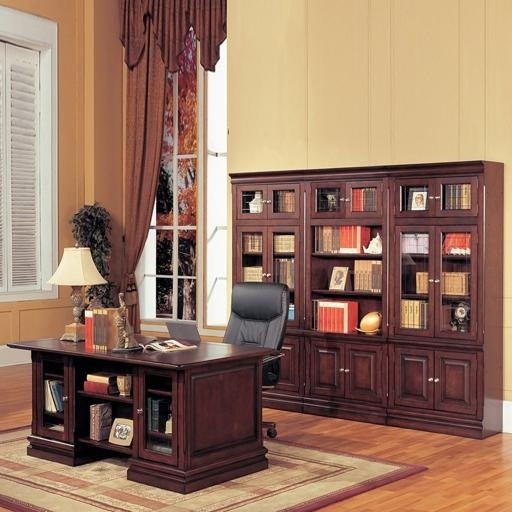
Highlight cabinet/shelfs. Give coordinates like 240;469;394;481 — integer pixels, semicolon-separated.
227;160;505;441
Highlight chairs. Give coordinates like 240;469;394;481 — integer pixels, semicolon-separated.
220;281;290;439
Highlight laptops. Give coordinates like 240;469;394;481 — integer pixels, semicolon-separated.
163;318;202;342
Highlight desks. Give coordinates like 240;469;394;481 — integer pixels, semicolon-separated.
5;337;278;497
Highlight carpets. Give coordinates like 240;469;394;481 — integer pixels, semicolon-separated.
0;424;429;511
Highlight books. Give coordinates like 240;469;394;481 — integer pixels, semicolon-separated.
94;403;112;441
87;371;117;384
90;405;95;440
243;184;471;336
147;397;168;432
84;381;119;394
147;441;172;454
85;308;129;351
45;380;64;413
138;336;197;352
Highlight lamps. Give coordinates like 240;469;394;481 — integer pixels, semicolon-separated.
46;246;109;343
